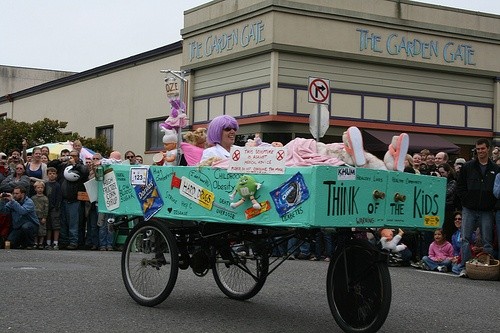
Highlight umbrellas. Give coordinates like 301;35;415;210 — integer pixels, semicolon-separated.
20;139;105;165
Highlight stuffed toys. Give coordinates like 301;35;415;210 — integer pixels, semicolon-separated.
229;174;262;209
181;128;207;148
382;226;406;252
159;98;187;133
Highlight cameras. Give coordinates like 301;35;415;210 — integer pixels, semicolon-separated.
1;193;9;197
13;156;18;160
65;156;72;161
432;173;437;176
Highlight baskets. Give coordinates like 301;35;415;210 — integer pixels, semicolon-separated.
466;252;500;280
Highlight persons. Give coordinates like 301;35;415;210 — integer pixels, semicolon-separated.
161;134;179;167
0;138;500;277
199;116;409;172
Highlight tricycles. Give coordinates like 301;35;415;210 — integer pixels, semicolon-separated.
95;159;448;333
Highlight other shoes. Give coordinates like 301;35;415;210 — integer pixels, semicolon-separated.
98;246;114;250
45;243;59;250
59;242;80;250
459;270;468;278
32;243;44;249
438;265;448;273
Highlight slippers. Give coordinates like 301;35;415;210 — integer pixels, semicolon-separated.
389;133;410;172
344;126;367;166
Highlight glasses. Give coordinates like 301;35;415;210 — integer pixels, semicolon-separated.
93;158;100;160
125;156;134;159
453;218;462;220
223;127;237;132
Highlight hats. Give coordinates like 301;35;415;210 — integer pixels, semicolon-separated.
455;158;466;164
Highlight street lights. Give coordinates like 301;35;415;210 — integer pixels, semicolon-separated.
159;69;186;167
